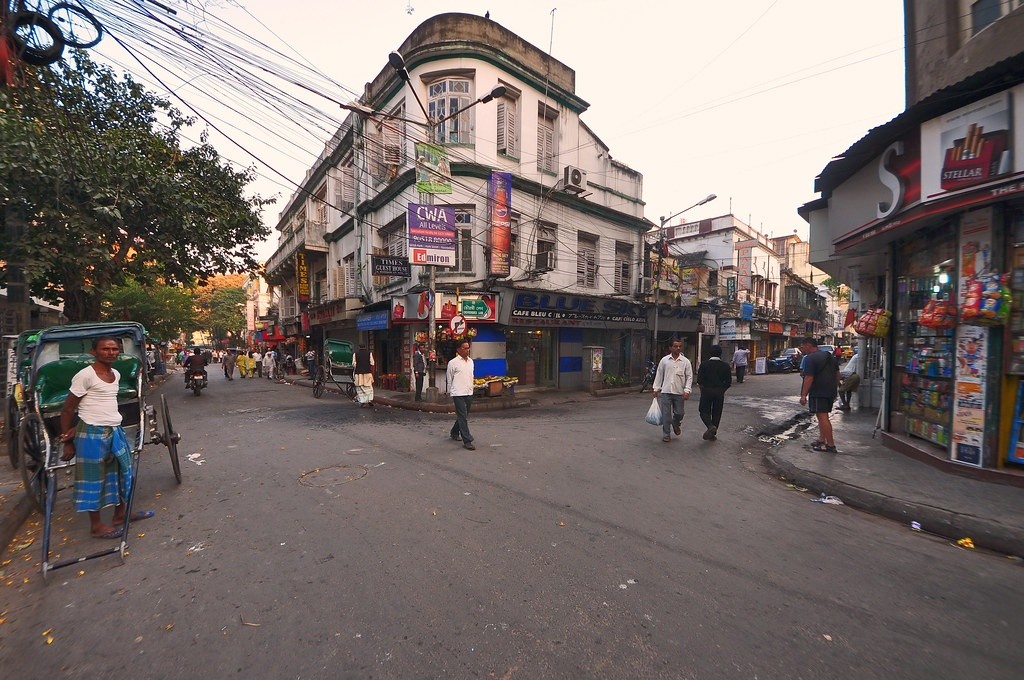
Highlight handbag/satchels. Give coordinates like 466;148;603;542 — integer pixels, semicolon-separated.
645;397;663;426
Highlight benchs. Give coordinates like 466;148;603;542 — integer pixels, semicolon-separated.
37;354;141;418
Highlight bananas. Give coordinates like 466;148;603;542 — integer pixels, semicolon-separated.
474;376;518;384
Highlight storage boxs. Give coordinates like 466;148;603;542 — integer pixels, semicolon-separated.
503;385;515;394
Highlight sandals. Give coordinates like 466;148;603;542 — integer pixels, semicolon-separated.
811;439;825;446
813;444;837;453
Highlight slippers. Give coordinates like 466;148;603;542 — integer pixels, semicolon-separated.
92;527;122;538
129;511;153;520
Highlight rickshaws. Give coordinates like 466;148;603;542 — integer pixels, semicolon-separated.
4;329;44;471
313;338;374;408
16;319;182;584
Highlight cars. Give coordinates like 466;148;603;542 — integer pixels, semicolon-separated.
817;345;837;355
767;347;804;373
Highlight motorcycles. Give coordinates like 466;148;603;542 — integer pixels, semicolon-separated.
639;360;658;394
182;365;209;397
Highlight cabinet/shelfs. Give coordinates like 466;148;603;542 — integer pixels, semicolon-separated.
899;273;956;449
487;380;503;396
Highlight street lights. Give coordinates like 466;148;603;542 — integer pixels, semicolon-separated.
650;193;717;383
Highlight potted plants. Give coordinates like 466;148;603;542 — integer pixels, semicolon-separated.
396;373;411;393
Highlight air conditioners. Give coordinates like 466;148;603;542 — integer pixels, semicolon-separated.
759;306;772;316
536;252;555;271
637;276;654;294
564;165;587;192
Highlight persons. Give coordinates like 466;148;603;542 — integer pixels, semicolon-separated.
351;343;376;407
59;335;154;539
799;337;860;453
697;345;732;441
181;348;292;389
305;346;316;380
652;339;693;442
447;339;475;450
732;345;750;383
413;343;427;402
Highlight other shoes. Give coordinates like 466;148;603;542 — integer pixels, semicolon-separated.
464;440;476;450
663;436;670;442
702;426;717;441
672;422;681;435
449;433;462;441
710;436;717;441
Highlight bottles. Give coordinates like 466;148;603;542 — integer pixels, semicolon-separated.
896;276;907;294
895;321;905;366
900;385;951;408
907;416;948;444
905;351;952;378
910;323;954;336
909;337;954;350
911;278;954;291
492;177;510;274
904;399;949;424
908;304;923;320
902;373;952;392
